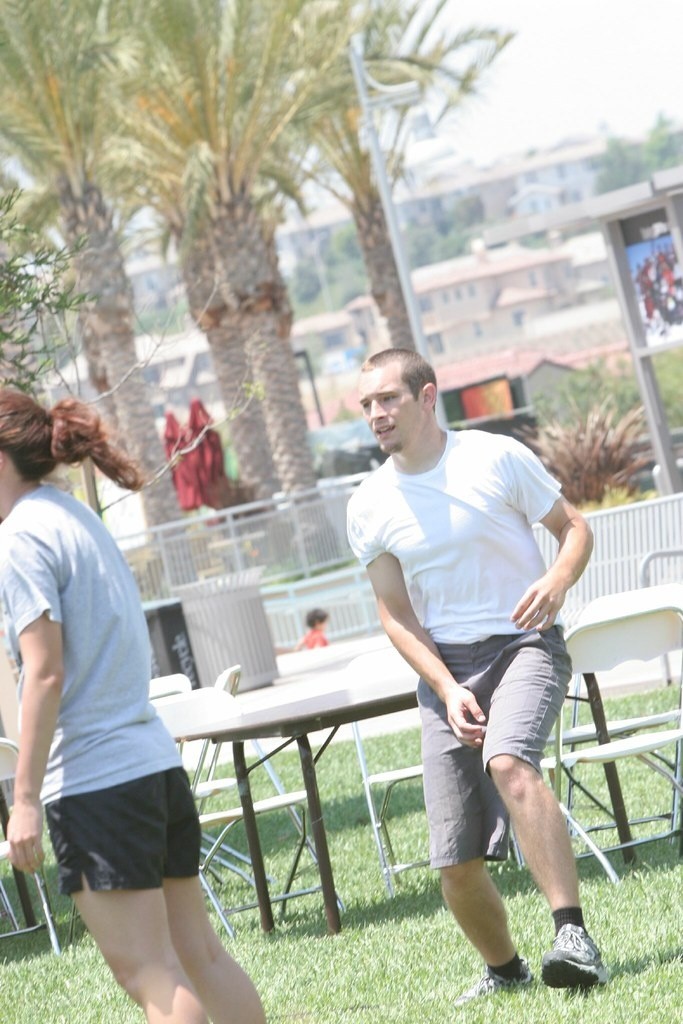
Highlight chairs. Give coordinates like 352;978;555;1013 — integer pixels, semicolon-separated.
1;549;683;957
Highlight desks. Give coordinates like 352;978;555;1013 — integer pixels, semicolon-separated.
169;674;433;935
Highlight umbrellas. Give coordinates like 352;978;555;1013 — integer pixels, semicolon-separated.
164;396;230;517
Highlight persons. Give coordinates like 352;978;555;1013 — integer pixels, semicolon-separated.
346;347;610;1003
1;388;266;1024
297;608;329;649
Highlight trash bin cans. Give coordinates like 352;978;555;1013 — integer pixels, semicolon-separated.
170;564;282;693
440;371;540;454
139;598;202;692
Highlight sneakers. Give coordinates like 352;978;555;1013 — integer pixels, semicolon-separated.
541;923;608;985
455;959;532;1006
